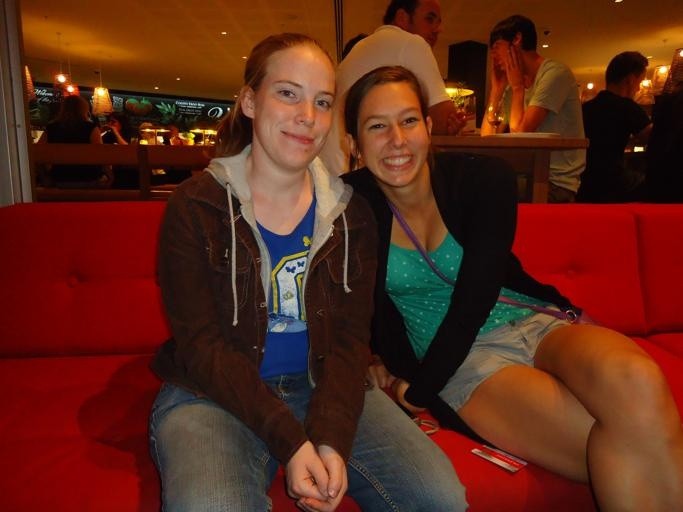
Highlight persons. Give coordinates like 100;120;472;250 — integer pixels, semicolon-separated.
642;47;683;202
33;92;193;191
330;1;465;179
333;67;682;512
582;49;654;204
146;33;468;511
481;15;587;204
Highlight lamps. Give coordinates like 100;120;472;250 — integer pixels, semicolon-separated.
54;56;114;116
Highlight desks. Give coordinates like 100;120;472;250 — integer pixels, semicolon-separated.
428;132;590;203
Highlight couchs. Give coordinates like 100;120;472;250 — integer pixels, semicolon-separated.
0;203;683;512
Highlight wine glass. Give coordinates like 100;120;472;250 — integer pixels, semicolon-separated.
485;99;506;135
